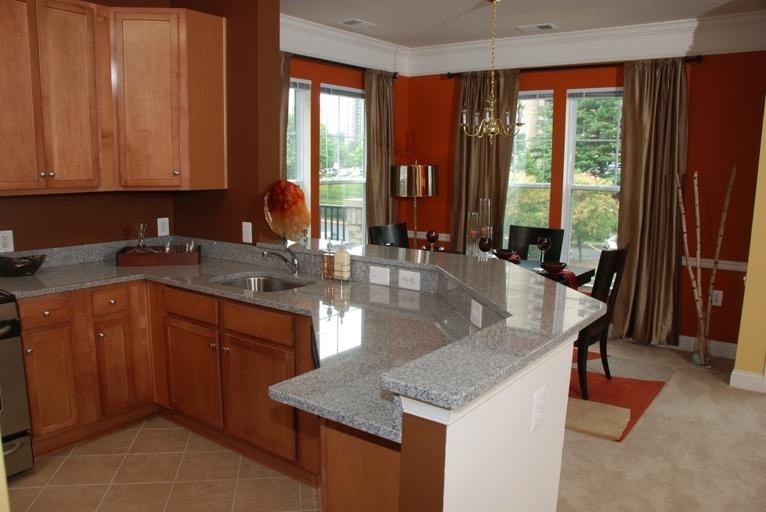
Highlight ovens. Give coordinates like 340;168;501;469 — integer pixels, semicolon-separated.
0;319;36;481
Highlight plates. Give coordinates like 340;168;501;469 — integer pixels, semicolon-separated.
264;179;315;241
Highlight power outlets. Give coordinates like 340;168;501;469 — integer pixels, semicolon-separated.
0;229;15;253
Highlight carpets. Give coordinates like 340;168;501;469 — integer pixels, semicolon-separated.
564;349;676;444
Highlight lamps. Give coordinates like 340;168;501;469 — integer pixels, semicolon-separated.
457;0;527;147
391;159;438;249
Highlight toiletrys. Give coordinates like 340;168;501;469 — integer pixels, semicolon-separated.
322;239;351;281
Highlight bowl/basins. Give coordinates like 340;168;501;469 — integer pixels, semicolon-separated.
2;252;48;276
540;262;567;274
491;248;515;260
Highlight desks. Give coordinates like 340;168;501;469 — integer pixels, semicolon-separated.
427;250;596;288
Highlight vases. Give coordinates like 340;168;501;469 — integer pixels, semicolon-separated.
689;307;715;368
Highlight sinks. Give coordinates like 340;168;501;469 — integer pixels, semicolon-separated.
220;276;306;293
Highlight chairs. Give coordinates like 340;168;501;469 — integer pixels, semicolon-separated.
508;225;564;262
572;248;630;400
368;222;409;249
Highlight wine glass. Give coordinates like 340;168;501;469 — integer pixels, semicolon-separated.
538;235;550;273
130;221;196;254
423;229;441;251
480;236;492;259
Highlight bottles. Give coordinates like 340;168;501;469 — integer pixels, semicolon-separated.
321;237;350;280
467;196;493;259
321;280;351;325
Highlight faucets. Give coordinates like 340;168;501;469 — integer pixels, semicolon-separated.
262;247;300;276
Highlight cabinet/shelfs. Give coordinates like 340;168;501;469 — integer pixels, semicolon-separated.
17;291;94;456
82;278;156;438
109;6;227;193
0;0;115;197
146;280;312;484
